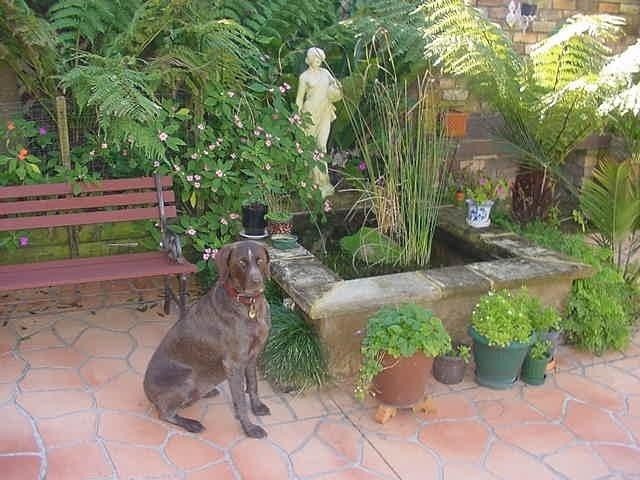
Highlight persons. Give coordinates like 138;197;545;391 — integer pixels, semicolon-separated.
295;46;343;198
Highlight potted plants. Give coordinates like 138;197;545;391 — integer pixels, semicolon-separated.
355;286;562;410
437;107;468;140
466;198;495;229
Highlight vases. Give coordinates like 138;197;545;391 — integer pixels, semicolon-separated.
244;202;266;235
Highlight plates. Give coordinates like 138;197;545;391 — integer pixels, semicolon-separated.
239;230;272;239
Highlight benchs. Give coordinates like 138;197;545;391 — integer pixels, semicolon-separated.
0;166;200;324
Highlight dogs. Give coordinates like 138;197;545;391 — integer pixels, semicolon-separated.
143;240;272;439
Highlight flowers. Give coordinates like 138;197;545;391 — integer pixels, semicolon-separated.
460;166;514;203
189;96;328;219
